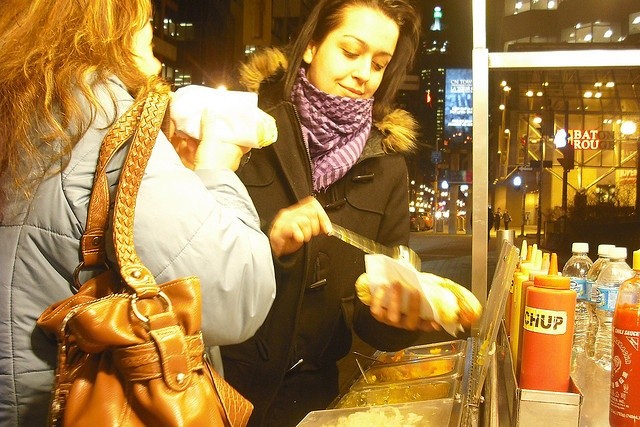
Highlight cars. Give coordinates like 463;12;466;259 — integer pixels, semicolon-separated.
409;212;424;232
419;213;432;229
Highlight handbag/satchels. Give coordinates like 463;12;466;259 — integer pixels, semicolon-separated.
37;269;254;427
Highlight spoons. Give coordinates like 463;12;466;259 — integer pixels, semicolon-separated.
329;224;423;272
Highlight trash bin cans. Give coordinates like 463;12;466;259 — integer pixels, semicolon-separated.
544;220;559;251
437;218;443;232
497;230;514;257
457;215;465;231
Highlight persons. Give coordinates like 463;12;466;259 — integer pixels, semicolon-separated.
493;207;503;231
0;0;277;427
222;0;445;427
470;200;494;251
502;209;512;230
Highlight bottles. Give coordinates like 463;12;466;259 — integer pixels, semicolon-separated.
562;241;593;354
586;243;616;360
595;247;634;371
506;239;578;391
608;248;640;426
